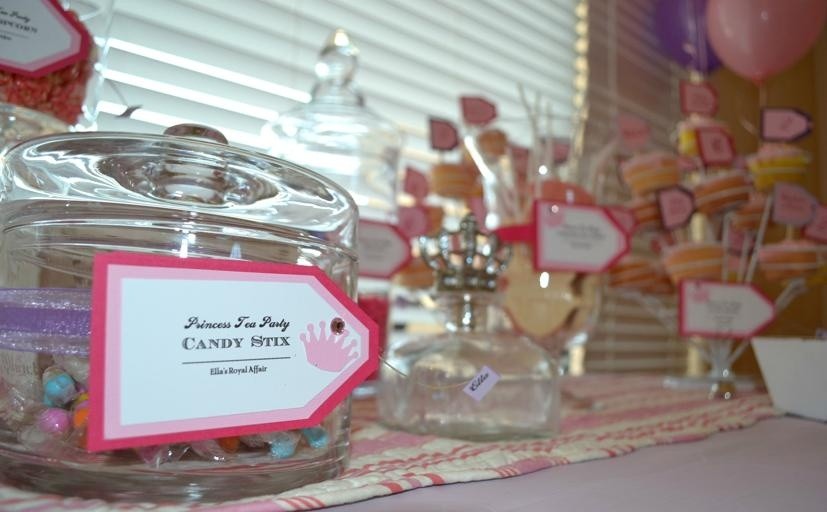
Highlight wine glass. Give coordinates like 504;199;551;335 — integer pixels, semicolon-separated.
499;251;604;411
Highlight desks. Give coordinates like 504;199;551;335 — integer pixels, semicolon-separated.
0;371;827;511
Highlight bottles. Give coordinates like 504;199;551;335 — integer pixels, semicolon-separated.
376;289;561;439
1;120;362;497
